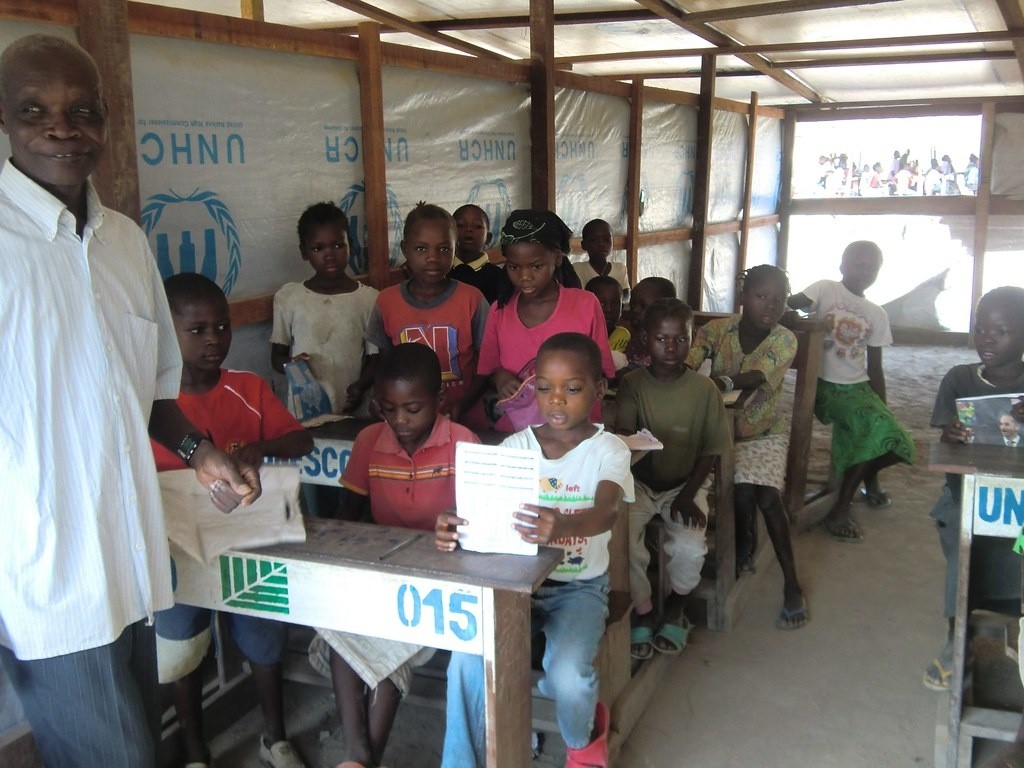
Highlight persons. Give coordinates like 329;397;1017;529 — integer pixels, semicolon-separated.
682;264;811;633
565;216;632;307
268;199;388;420
781;240;916;544
617;276;684;374
366;200;496;429
616;300;740;666
0;35;263;768
472;208;618;435
308;341;484;768
795;149;980;197
921;287;1024;696
433;331;636;768
994;413;1024;445
400;201;506;304
581;274;631;369
146;269;316;768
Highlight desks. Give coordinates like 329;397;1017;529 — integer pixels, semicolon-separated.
923;437;1023;768
154;312;843;768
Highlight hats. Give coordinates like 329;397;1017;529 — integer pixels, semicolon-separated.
502;208;573;255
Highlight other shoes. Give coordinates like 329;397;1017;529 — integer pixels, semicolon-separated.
261;735;306;768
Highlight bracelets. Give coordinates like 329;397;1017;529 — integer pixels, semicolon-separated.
718;375;734;393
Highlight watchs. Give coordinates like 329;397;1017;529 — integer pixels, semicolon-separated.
177;427;213;468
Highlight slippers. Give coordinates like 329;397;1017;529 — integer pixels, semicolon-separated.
820;517;864;543
735;557;755;574
861;486;892;509
653;616;692;656
562;701;609;768
631;617;653;659
778;592;808;629
924;652;975;689
